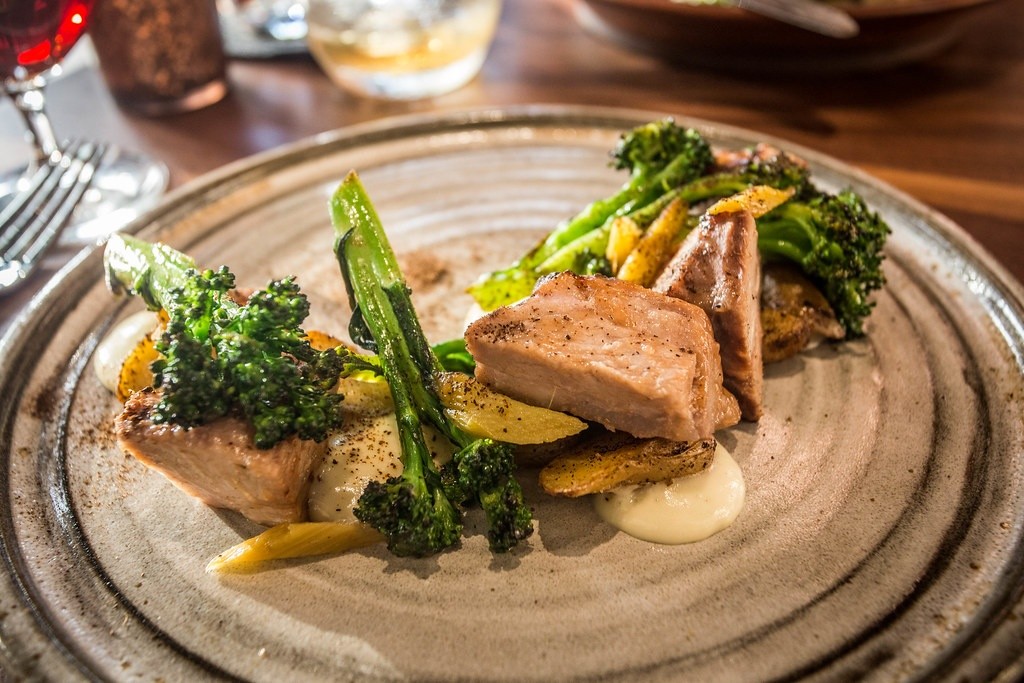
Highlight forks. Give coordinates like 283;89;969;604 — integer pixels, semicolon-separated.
2;141;108;295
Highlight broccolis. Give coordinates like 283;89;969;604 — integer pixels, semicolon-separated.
524;122;890;337
102;232;343;447
328;170;531;556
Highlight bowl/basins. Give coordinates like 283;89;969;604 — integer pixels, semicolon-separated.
584;0;985;49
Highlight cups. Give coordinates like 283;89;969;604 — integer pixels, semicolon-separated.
308;1;502;101
84;1;232;117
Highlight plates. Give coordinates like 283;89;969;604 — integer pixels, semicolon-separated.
568;1;979;71
2;102;1024;682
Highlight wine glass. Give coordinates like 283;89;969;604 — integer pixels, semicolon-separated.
2;0;171;250
